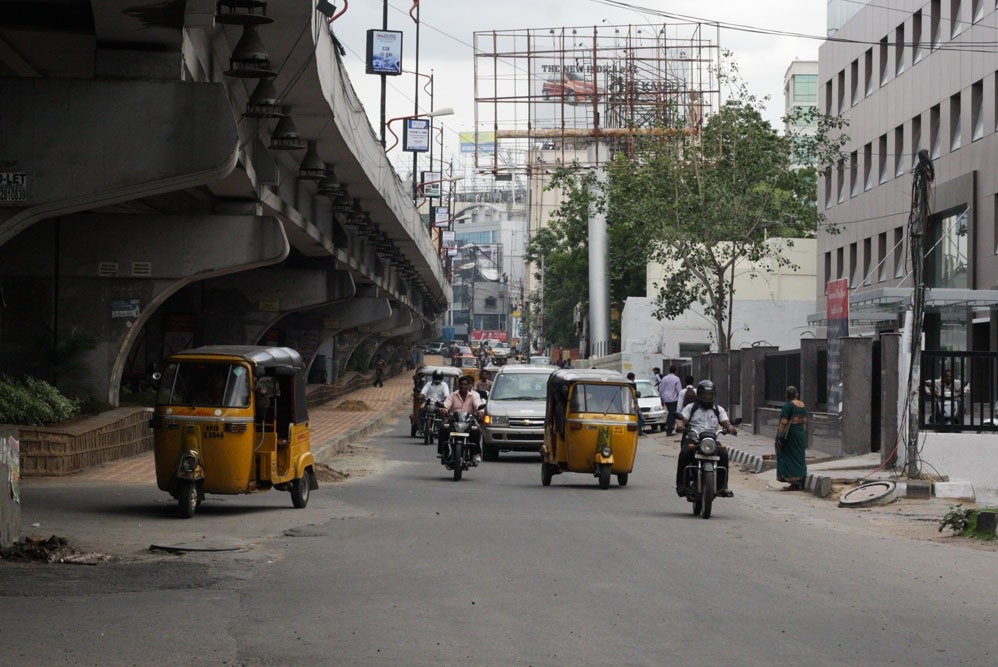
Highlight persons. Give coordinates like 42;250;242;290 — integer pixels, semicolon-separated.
627;372;647;436
924;369;971;397
406;336;575;464
776;386;808;491
659;366;684;436
373;354;387;387
652;367;665;392
195;372;243;408
675;380;738;498
578;386;619;413
675;375;698;423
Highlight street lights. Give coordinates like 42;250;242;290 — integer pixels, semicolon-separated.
386;108;456;152
432;215;471;241
417;175;466;209
441;243;473;266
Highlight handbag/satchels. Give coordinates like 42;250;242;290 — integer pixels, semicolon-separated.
779;402;795;441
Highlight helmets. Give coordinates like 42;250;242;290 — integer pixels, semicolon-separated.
696;379;716;408
432;370;443;380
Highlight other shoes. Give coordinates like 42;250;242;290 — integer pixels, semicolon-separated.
667;433;676;436
639;432;646;436
782;484;804;491
720;490;734;497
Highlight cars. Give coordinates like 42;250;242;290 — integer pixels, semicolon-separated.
481;366;563;462
430;336;552;388
542;73;603;105
634;379;669;429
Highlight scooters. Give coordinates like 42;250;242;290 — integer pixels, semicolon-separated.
926;380;971;427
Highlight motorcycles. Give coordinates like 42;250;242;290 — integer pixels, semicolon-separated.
435;401;485;481
418;394;444;443
147;344;318;519
676;411;743;521
540;370;640;490
409;365;464;439
